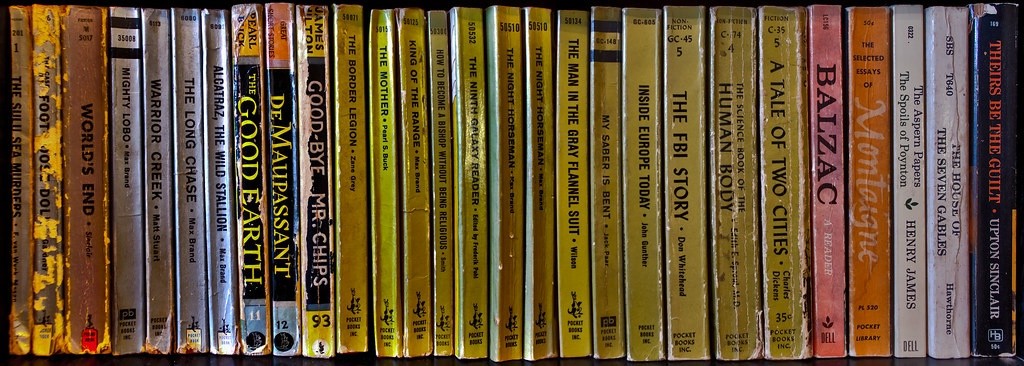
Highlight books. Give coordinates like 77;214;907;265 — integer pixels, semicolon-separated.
8;2;1021;363
